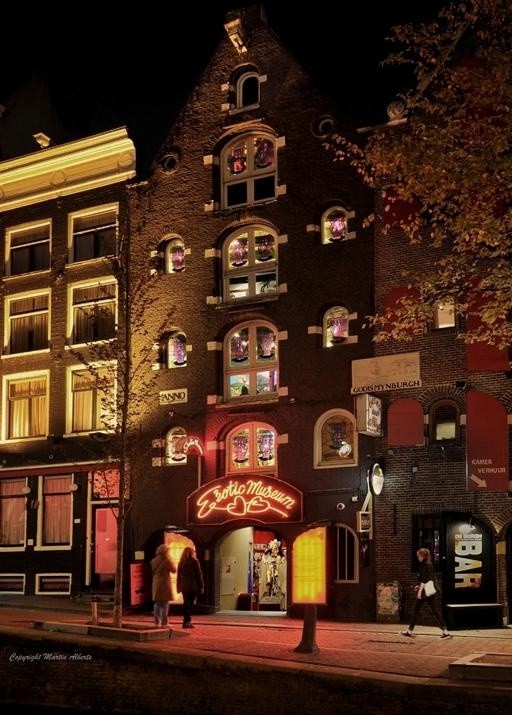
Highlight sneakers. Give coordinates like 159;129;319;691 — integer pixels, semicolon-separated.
440;633;449;639
400;630;413;637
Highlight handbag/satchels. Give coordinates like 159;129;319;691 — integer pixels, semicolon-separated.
417;579;439;599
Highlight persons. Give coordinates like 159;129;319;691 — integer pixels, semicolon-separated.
149;544;177;628
403;545;453;637
176;546;206;629
